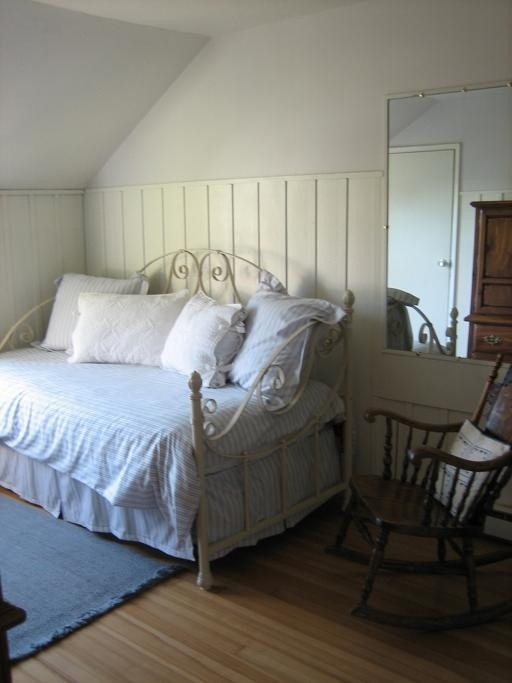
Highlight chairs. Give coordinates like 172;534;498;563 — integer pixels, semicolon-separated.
329;355;511;630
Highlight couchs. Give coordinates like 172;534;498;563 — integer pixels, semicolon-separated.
0;248;356;591
386;289;447;354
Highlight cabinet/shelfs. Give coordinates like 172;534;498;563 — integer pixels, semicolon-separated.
468;197;512;360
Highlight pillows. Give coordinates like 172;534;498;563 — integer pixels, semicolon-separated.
69;291;191;367
227;271;345;413
159;289;246;390
33;270;158;349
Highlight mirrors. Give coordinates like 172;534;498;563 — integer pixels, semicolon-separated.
384;79;510;360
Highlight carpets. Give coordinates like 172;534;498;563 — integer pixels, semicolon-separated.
0;491;193;666
